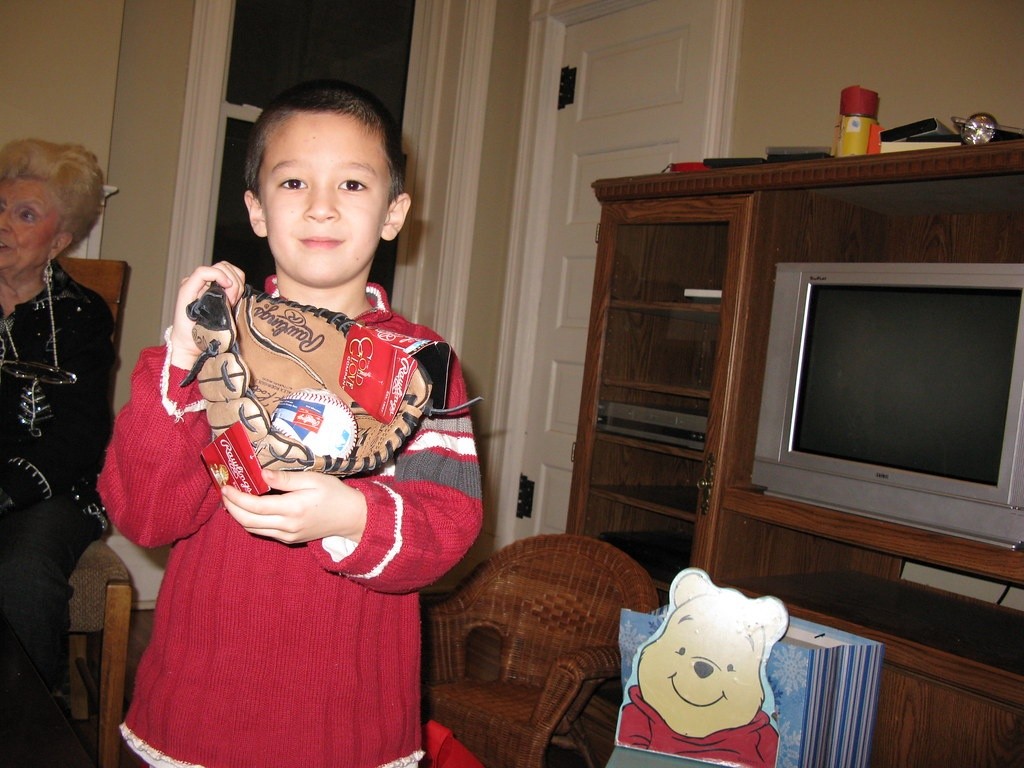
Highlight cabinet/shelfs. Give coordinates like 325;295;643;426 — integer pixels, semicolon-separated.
564;138;1024;768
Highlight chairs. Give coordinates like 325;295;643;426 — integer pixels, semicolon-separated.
56;257;134;768
420;533;660;768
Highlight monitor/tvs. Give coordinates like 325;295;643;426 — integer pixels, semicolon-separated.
750;264;1024;551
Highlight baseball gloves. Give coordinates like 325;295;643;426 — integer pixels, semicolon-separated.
178;280;486;477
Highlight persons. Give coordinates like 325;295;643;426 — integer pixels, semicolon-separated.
95;79;483;768
0;138;115;702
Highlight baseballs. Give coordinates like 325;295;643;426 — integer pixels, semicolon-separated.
270;388;358;461
